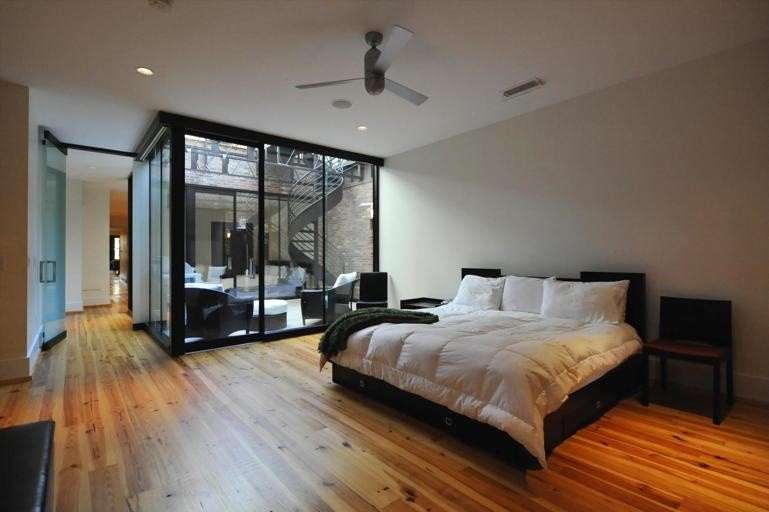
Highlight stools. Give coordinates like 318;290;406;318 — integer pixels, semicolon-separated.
0;420;55;512
249;299;288;331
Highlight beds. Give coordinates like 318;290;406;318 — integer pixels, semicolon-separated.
317;267;646;471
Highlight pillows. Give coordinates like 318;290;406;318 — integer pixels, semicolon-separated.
542;279;630;322
333;272;356;294
501;275;555;313
185;283;224;293
453;275;505;310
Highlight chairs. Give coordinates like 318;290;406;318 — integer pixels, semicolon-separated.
642;296;734;425
356;272;387;309
185;288;254;339
300;279;359;326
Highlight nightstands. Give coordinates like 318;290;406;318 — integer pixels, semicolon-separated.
400;297;445;309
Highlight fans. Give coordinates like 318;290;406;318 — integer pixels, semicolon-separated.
295;26;429;106
